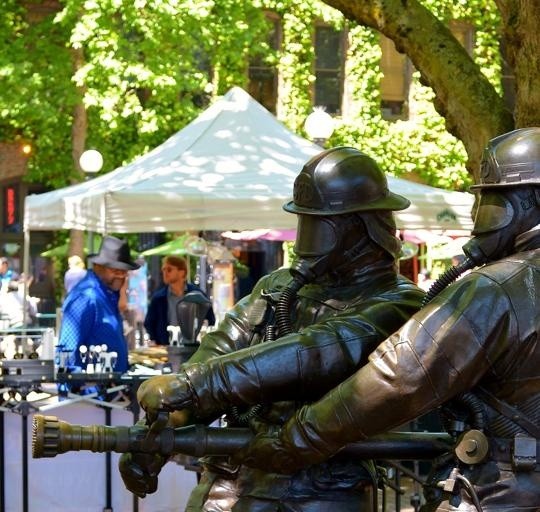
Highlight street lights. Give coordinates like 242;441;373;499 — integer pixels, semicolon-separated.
77;149;103;258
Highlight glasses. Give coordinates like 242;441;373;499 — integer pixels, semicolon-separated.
161;267;171;272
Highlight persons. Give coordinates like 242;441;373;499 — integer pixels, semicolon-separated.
227;126;540;512
0;235;216;402
119;147;426;512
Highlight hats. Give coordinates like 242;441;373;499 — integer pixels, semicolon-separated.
87;236;141;270
